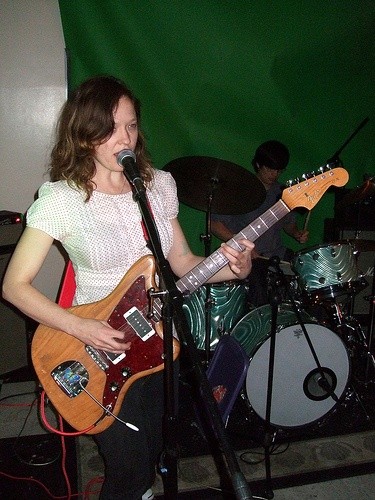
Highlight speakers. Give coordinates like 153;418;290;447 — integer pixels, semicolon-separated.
0;210;30;379
322;218;375;318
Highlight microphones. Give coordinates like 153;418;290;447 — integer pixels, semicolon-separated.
317;377;340;403
116;149;146;194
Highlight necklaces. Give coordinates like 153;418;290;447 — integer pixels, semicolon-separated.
93;178;126;194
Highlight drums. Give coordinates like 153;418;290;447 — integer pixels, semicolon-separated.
175;279;252;352
228;301;354;431
289;238;363;302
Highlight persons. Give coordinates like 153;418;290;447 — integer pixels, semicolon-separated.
211;139;308;311
1;76;256;500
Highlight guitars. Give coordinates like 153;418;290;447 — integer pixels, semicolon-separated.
27;163;355;439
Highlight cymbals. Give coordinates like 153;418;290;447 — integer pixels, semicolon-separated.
160;155;268;216
